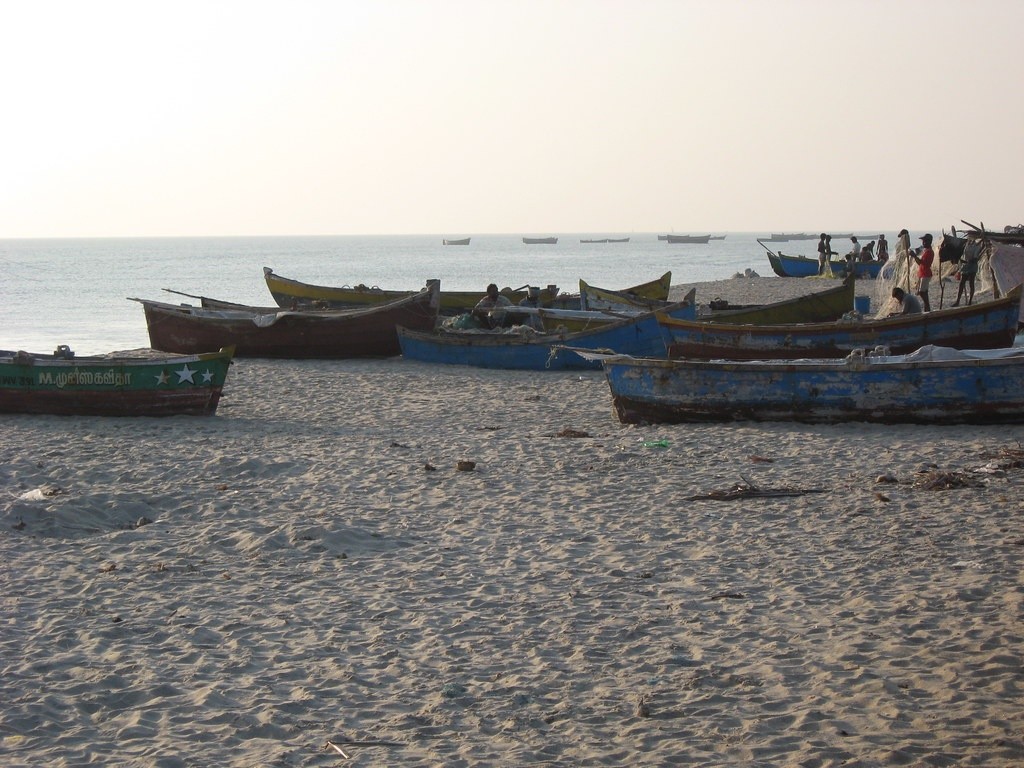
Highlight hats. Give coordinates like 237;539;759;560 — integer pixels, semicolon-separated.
920;234;932;242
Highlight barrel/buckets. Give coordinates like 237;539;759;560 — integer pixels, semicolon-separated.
855;295;870;318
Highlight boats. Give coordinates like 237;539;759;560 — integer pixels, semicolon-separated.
124;267;1024;359
517;233;884;244
765;251;897;278
442;237;472;246
544;343;1024;425
392;286;697;371
0;345;234;418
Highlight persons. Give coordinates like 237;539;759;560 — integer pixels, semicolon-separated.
849;237;875;262
817;233;838;275
950;237;982;306
909;233;934;312
519;287;545;331
846;254;855;276
472;285;513;329
894;229;908;286
876;235;889;262
889;287;921;315
